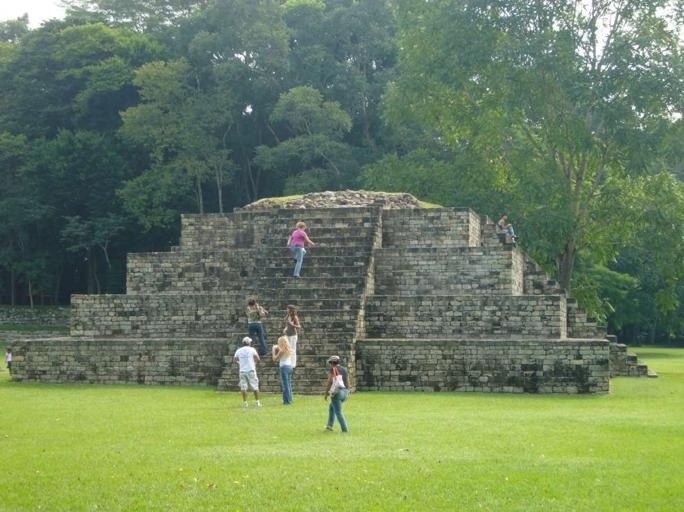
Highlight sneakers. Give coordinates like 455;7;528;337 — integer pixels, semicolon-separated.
242;400;262;409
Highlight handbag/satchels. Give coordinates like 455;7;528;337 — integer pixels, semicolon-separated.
328;368;346;397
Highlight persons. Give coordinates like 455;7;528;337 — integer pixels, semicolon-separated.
3;347;11;377
244;296;269;357
321;354;349;432
277;304;301;367
232;336;261;407
495;213;519;244
285;221;314;278
270;335;293;405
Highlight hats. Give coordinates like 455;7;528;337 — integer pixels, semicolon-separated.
286;304;297;310
327;356;341;364
242;337;253;346
